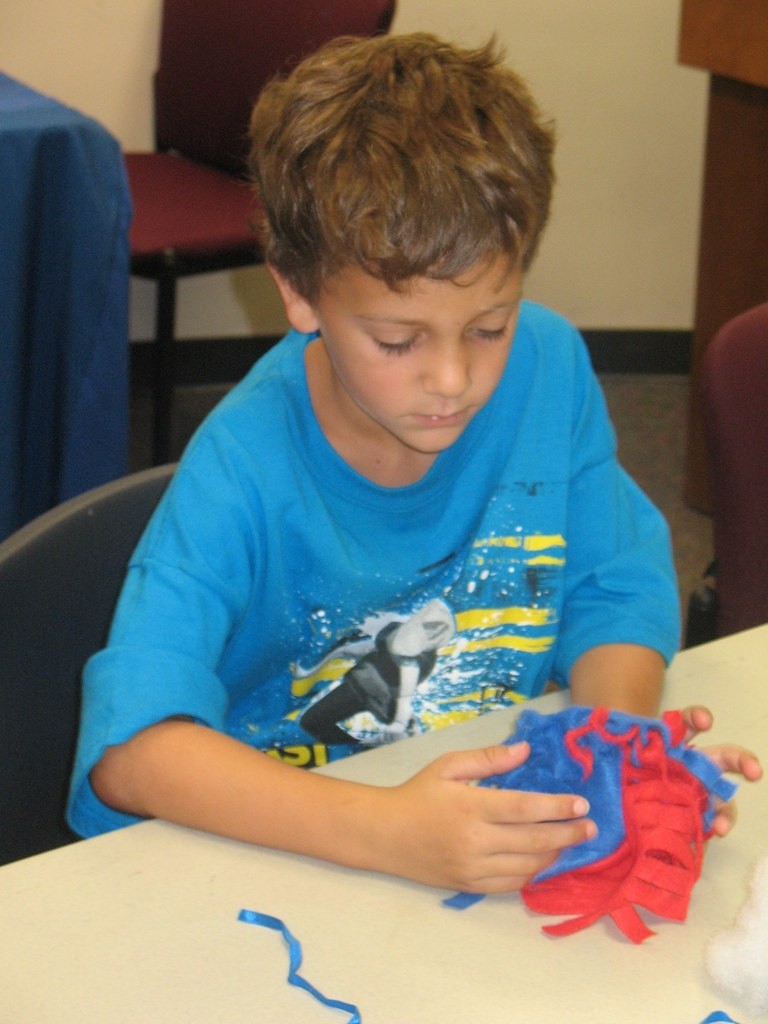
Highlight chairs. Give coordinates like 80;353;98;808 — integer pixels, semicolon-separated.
125;0;398;466
701;305;768;630
0;463;187;858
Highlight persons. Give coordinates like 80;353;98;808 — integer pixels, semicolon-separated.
66;30;762;893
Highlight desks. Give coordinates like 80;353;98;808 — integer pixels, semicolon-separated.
672;0;768;510
0;615;768;1024
0;71;133;542
